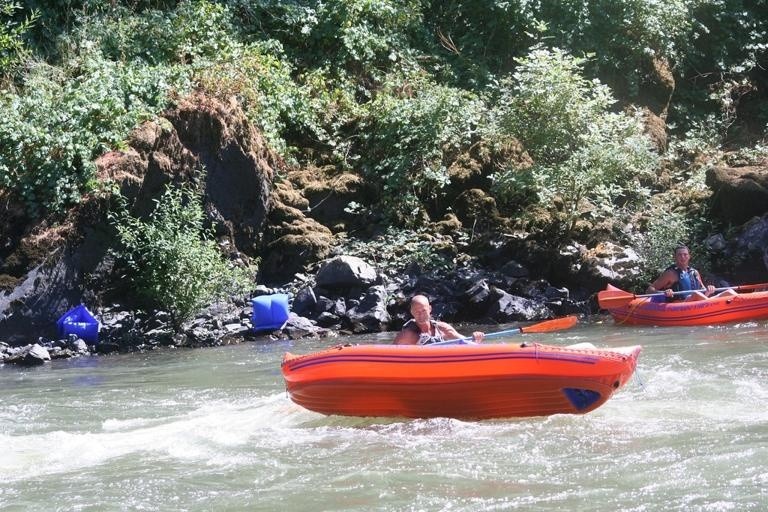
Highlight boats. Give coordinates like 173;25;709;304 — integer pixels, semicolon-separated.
601;282;767;328
278;344;641;420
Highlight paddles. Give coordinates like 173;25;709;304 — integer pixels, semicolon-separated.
599;284;768;309
426;315;577;347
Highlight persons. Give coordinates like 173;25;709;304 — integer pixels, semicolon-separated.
647;245;740;302
393;294;486;355
